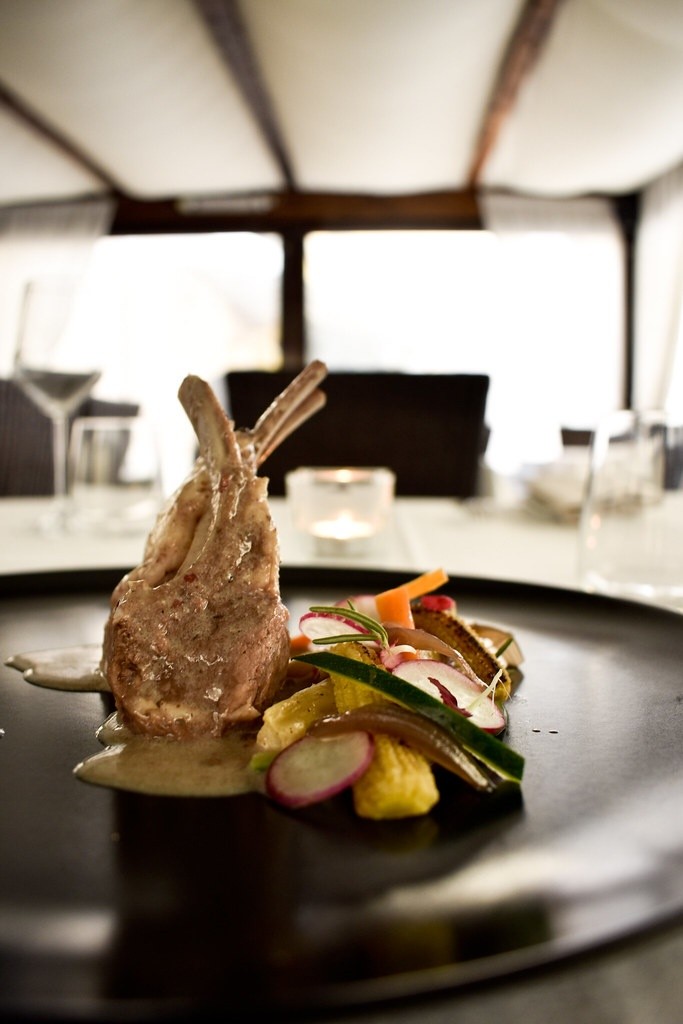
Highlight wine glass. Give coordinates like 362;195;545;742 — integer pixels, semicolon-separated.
10;275;106;543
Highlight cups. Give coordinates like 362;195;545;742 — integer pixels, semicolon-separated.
60;415;160;549
575;405;683;606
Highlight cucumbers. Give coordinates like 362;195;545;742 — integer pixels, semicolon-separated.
294;650;525;783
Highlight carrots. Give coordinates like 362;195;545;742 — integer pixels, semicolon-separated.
289;568;449;649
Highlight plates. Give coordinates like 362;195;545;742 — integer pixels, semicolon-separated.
0;565;683;1023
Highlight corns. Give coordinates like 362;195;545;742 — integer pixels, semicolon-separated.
323;641;439;820
406;605;510;700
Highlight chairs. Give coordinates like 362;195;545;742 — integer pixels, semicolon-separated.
226;370;490;503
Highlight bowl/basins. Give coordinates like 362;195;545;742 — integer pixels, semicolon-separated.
285;467;395;539
